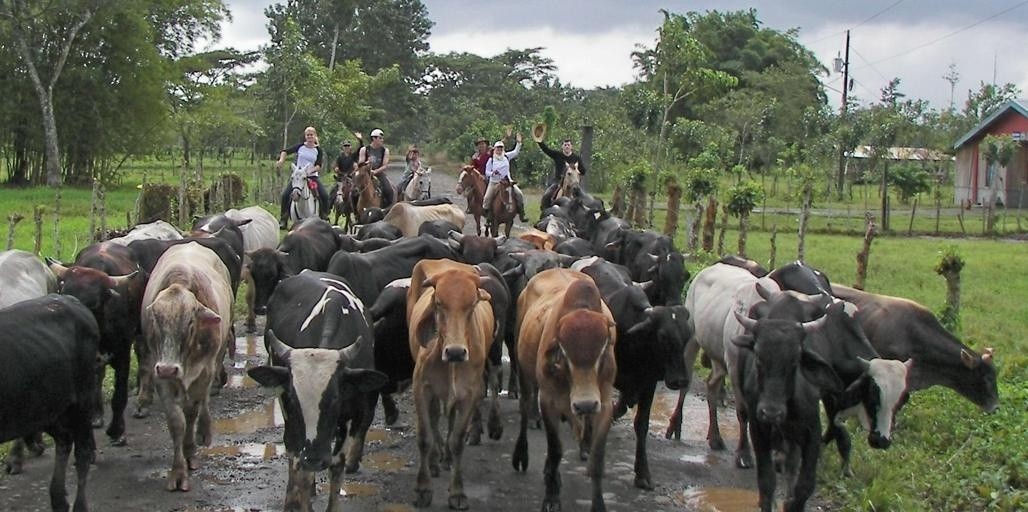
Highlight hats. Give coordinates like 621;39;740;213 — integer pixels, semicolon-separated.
371;129;384;138
531;122;547;143
494;141;504;148
473;136;489;145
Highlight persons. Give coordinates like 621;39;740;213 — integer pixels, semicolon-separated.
349;129;393;220
277;127;330;229
396;144;423;195
465;129;512;213
482;133;528;227
538;140;585;210
328;132;364;209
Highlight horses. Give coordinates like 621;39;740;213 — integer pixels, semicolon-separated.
403;166;432;201
456;163;488;237
544;160;582;200
351;162;397;215
289;161;320;225
329;176;353;233
490;178;518;237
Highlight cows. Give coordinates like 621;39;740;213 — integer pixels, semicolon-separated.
0;186;1000;512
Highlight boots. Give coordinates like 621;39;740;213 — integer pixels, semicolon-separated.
517;202;529;221
